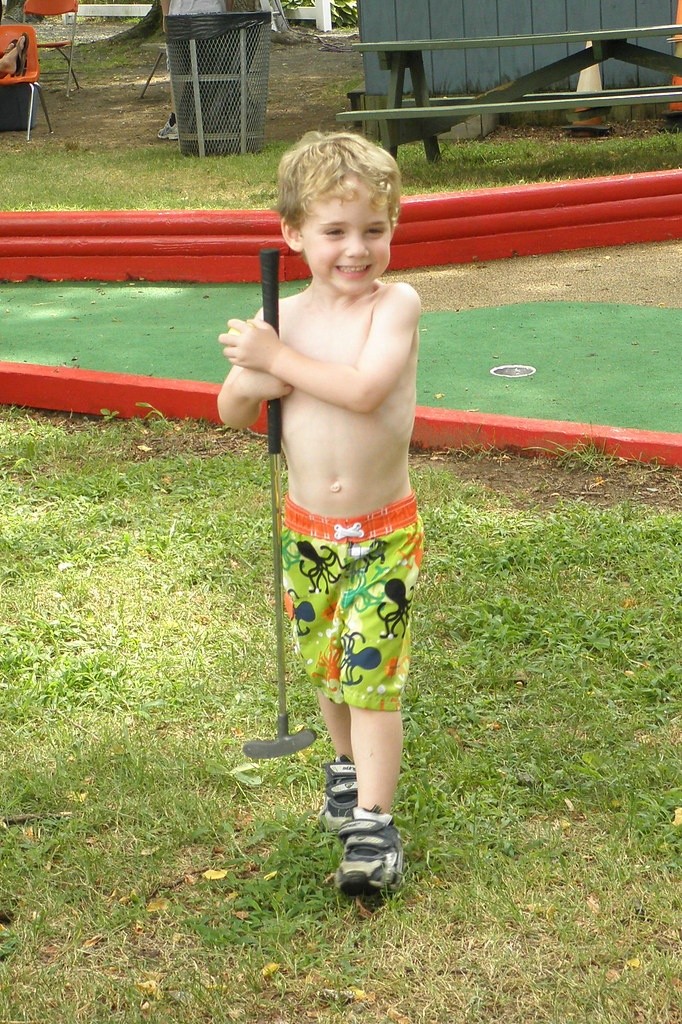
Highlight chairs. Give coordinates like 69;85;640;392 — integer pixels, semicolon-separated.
0;25;54;144
22;0;80;96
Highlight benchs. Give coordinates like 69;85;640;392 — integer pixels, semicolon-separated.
336;86;682;165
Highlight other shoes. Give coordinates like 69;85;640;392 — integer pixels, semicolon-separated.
157;114;178;139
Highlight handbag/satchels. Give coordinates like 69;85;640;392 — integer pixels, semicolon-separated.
0;32;29;79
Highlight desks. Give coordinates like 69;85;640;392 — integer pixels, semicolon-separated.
350;24;681;165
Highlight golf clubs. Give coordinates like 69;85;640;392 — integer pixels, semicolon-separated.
239;249;319;760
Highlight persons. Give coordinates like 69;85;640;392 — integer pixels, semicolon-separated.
0;31;30;79
218;130;426;895
157;0;234;139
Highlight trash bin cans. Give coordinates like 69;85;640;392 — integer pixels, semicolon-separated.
162;11;273;157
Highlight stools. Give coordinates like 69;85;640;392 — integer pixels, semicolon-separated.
140;42;169;99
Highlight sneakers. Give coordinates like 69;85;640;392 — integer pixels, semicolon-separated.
324;754;358;826
333;806;404;894
